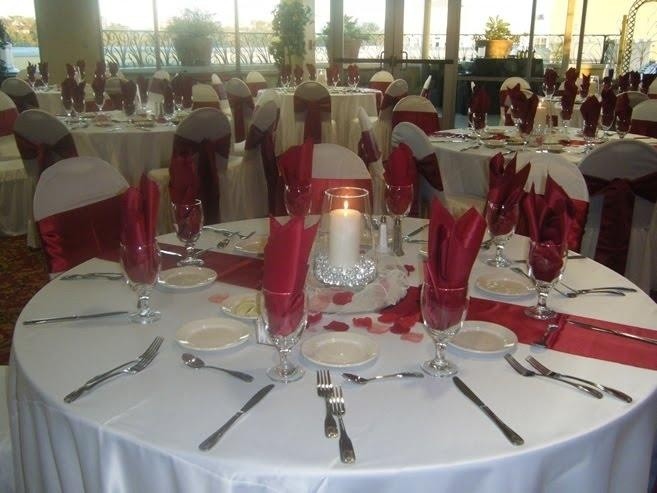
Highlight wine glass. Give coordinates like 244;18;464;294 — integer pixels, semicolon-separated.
60;92;193;127
521;238;569;321
169;198;206;267
416;280;471;377
538;74;652;101
283;182;314;219
463;104;631;151
25;66;120;92
383;182;413;257
117;238;165;326
481;198;521;268
257;283;310;387
277;67;360;94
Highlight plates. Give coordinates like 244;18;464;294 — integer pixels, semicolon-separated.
218;290;260;322
443;318;520;355
474;271;533;298
318;230;394;249
417;244;431;259
156;265;217;289
173;316;251;353
300;329;382;368
234;233;269;254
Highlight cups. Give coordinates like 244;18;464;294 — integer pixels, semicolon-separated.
310;183;376;286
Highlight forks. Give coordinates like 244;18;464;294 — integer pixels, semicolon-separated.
502;350;633;407
553;281;636;299
314;367;357;464
203;223;255;239
60;333;164;402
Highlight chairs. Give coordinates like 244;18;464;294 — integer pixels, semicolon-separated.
0;59;657;337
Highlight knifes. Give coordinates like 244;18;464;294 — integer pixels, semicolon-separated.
512;253;585;264
452;374;528;448
567;318;657;346
21;308;130;328
406;222;429;238
196;381;277;456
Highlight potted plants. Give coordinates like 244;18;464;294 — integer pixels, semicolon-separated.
321;15;362;63
485;15;513;59
165;8;226;66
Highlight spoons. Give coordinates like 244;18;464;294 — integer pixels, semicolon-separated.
179;353;254;384
341;370;422;385
531;322;557;349
192;236;229;257
60;269;125;282
